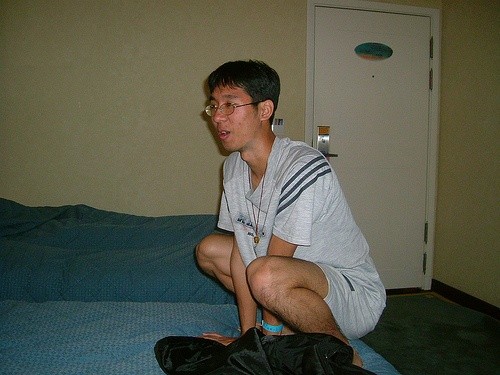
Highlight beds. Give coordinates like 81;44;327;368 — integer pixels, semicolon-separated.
0;198;400;375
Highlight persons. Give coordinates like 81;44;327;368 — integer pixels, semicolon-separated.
194;59;388;367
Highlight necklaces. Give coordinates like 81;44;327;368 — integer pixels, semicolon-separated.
242;151;275;246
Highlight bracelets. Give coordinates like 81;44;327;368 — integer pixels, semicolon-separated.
261;320;285;332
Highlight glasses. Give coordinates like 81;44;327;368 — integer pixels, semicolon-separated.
205;101;262;116
